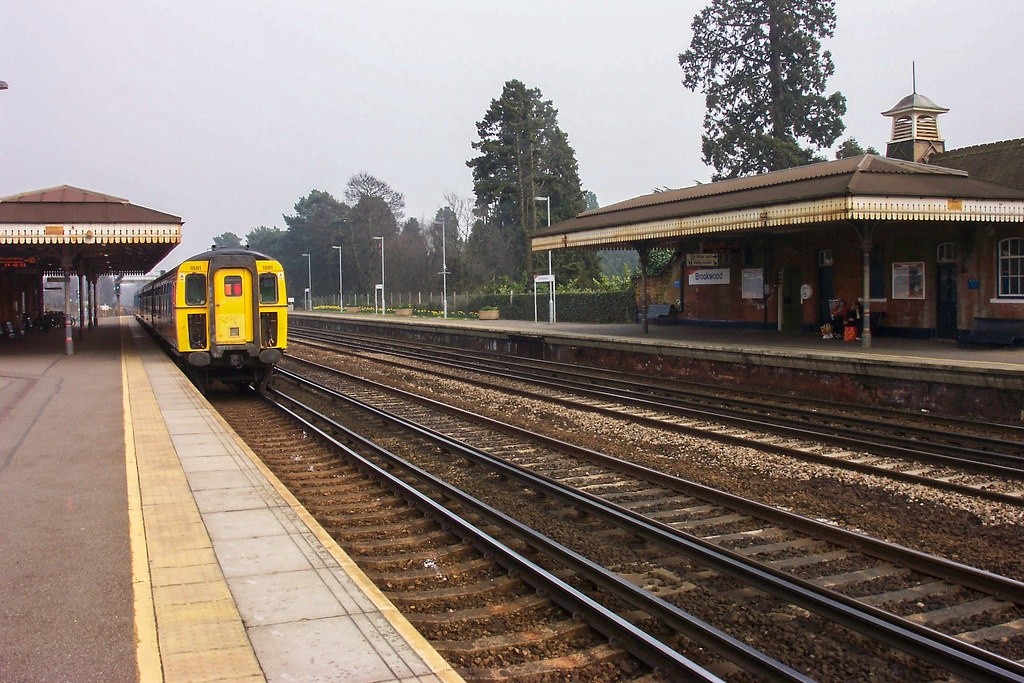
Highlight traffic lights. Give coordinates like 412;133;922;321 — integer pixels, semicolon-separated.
116;285;119;296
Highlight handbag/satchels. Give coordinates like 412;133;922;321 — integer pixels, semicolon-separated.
844;326;859;342
821;323;834;340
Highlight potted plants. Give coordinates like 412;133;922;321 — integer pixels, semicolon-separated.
396;305;412;316
479;306;499;320
346;304;359;313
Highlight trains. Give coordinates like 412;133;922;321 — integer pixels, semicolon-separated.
132;246;287;396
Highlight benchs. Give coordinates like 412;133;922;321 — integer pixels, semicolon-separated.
634;302;676;325
842;311;886;339
955;317;1024;347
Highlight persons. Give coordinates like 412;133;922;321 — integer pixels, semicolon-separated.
833;300;862;341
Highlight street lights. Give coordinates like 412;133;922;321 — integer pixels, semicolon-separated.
434;221;447;318
372;236;387;315
533;195;554;323
301;254;312;313
331;246;343;313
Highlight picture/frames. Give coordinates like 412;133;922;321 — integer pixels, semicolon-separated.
891;261;926;300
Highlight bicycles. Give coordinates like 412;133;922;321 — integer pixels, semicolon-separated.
36;310;77;331
